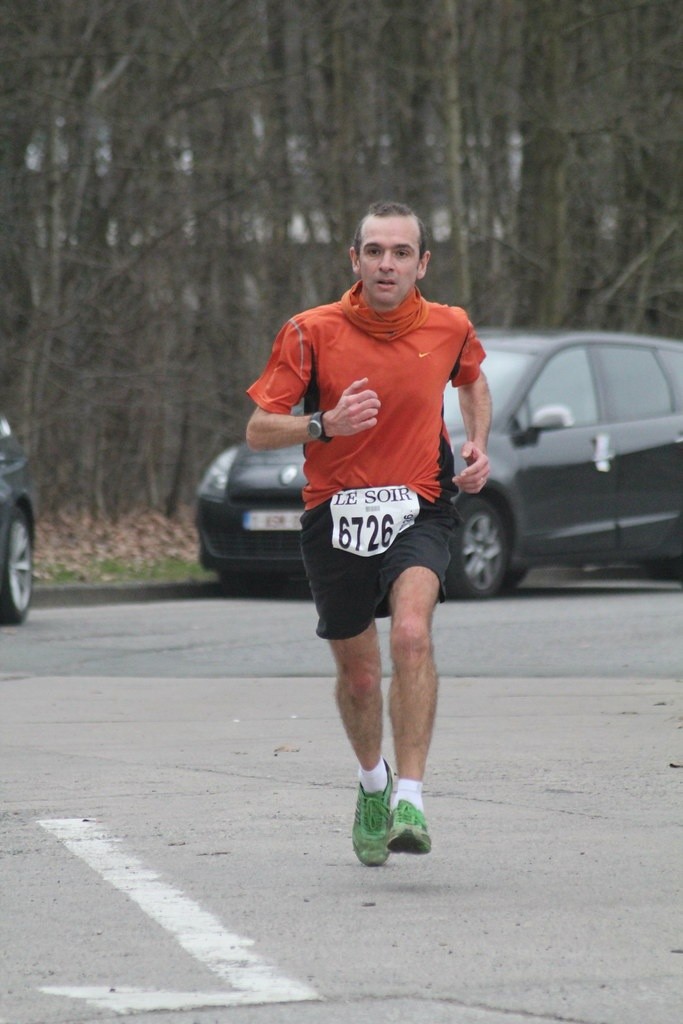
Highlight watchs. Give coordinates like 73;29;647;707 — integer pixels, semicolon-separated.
305;411;335;443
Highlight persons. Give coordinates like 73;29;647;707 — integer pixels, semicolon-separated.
246;201;495;859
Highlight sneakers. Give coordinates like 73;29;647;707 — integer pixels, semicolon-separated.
352;758;394;867
386;800;431;853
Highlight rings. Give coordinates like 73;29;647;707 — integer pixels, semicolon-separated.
482;477;485;485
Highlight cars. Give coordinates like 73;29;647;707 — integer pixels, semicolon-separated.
0;410;38;628
195;325;683;604
